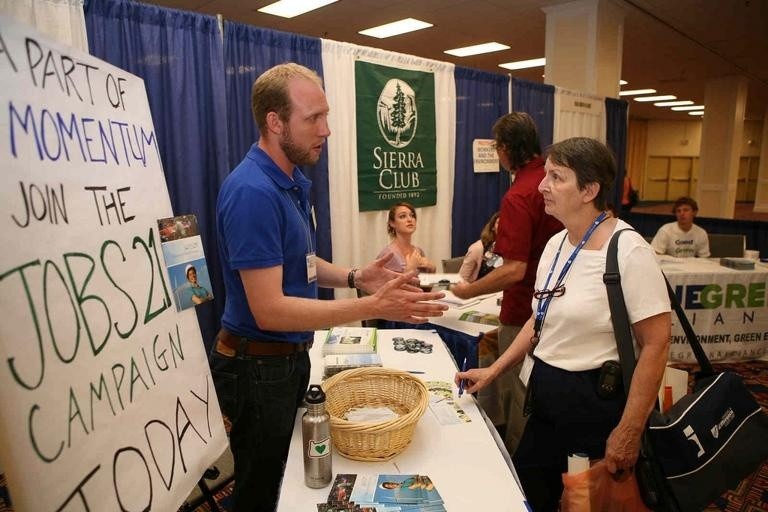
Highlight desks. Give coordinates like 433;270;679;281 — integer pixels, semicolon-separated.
384;272;504;399
630;257;767;366
275;328;533;512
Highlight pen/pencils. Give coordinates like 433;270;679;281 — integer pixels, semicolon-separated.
406;370;426;375
458;357;466;399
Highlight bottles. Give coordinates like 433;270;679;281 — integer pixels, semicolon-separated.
663;384;674;412
299;383;333;489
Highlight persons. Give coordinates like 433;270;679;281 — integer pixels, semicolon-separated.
211;60;449;512
451;111;567;461
378;477;417;491
185;264;212;306
649;196;711;259
375;203;468;369
454;136;671;512
459;211;505;286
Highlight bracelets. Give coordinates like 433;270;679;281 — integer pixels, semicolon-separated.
347;268;359;289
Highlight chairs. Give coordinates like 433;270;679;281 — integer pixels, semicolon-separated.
343;267;383;329
707;233;746;258
439;255;464;273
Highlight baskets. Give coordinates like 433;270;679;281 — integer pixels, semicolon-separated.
316;368;428;461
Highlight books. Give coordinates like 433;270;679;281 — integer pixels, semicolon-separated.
321;326;382;380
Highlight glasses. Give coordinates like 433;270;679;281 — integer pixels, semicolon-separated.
534;285;565;299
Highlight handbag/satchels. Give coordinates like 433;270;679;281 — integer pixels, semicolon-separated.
636;370;766;510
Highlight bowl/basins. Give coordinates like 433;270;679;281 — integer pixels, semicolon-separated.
419;284;432;292
